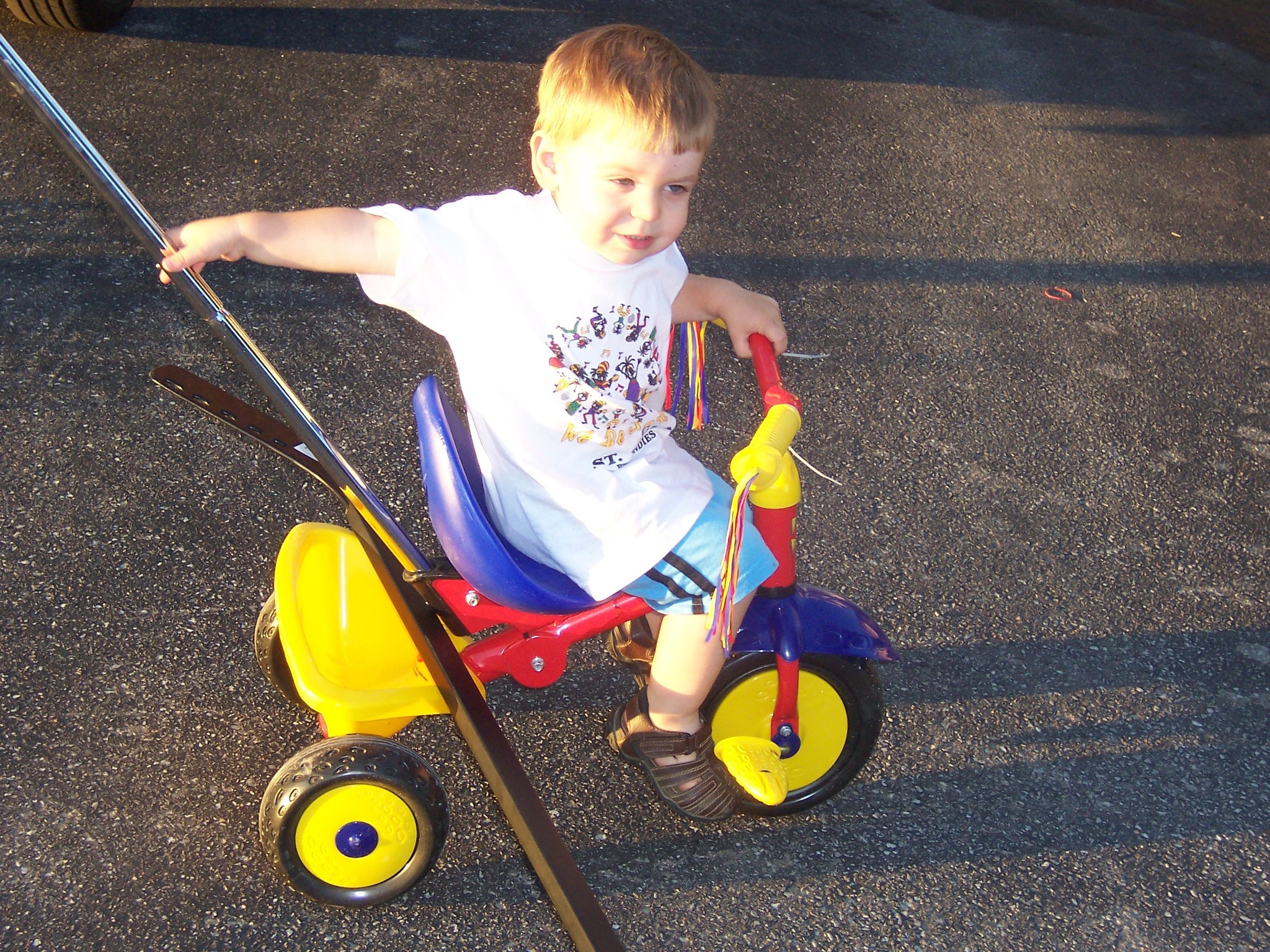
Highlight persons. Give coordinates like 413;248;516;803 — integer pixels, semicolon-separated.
153;23;788;823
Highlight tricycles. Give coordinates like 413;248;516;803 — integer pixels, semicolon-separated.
150;316;901;909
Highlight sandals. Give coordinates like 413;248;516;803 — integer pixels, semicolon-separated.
605;687;737;821
600;615;657;686
